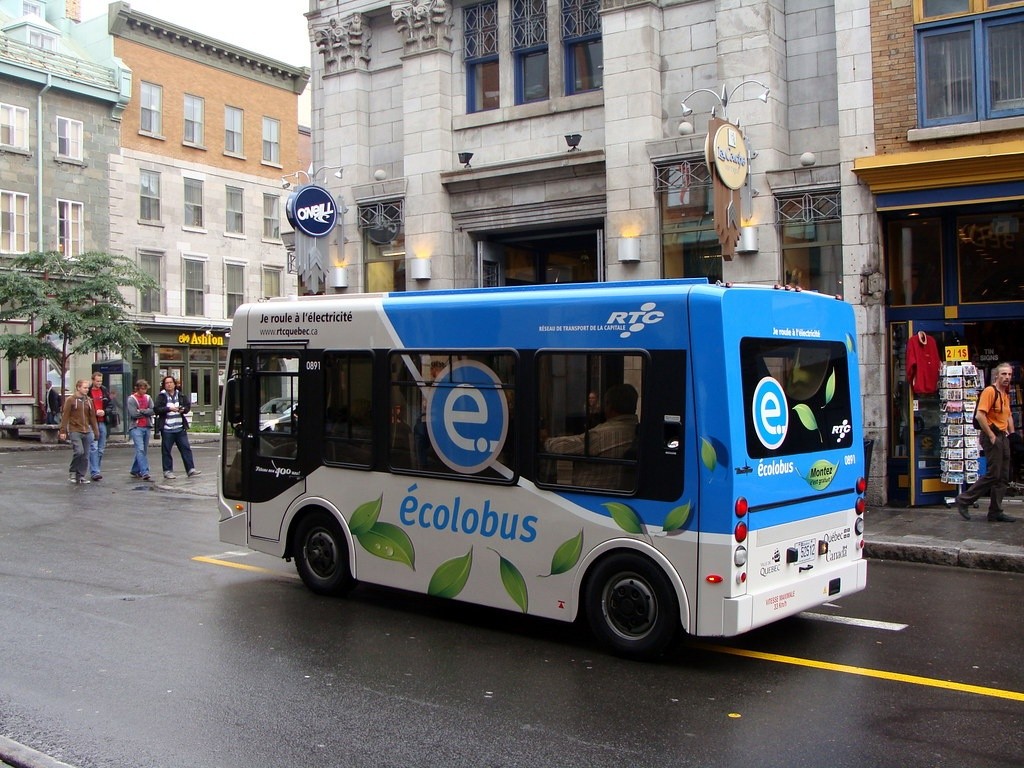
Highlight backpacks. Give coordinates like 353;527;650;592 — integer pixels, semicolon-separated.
972;385;998;430
58;395;61;407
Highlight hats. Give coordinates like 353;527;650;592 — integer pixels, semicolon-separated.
46;381;52;384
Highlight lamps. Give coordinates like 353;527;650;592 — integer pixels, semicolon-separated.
616;237;641;263
281;165;343;187
565;134;582;152
200;323;213;335
679;80;769;121
735;226;759;252
327;268;348;288
411;258;432;280
223;326;232;337
458;153;474;169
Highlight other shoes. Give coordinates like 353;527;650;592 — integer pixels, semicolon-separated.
70;473;77;483
136;472;143;477
79;478;90;484
142;475;155;482
92;475;102;480
988;512;1015;522
956;498;971;519
188;470;202;478
164;471;176;479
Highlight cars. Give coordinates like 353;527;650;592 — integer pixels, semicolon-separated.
259;397;299;435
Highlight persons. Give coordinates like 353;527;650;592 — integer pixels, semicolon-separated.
956;362;1018;522
430;362;446;378
88;372;113;479
128;379;155;480
413;401;430;471
155;376;201;478
59;379;99;484
544;384;640;488
45;381;59;424
589;391;600;414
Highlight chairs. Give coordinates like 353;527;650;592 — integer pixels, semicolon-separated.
619;419;684;492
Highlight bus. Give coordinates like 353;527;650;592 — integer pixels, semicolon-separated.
216;275;873;657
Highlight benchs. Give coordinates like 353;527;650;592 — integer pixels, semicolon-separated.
0;425;63;444
